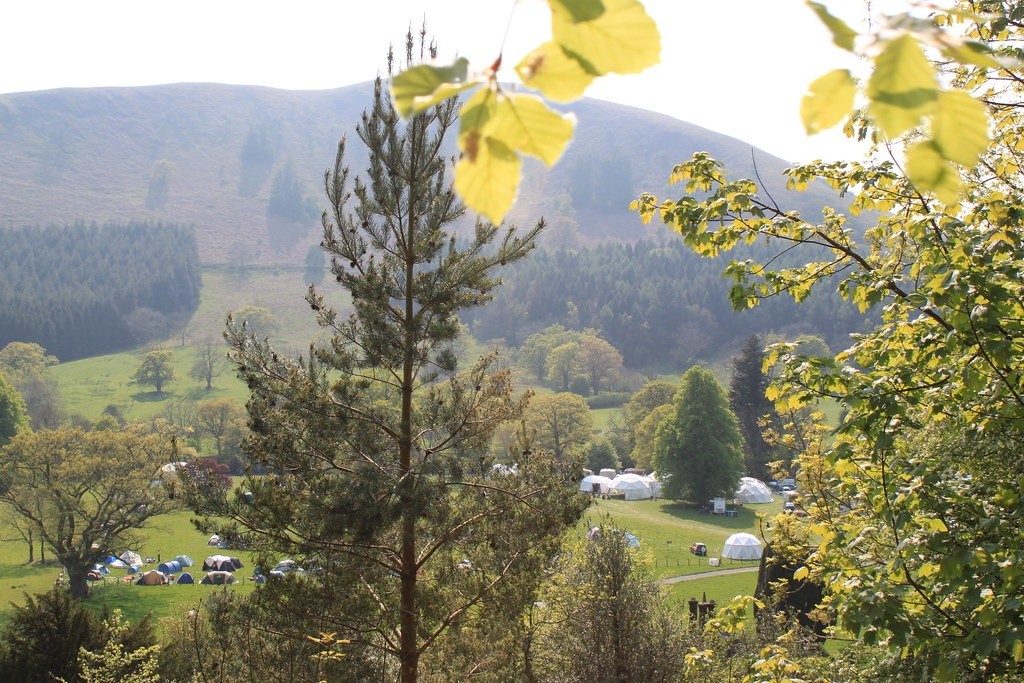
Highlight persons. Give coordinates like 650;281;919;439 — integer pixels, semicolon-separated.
157;549;161;561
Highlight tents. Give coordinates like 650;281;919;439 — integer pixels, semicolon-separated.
86;531;318;585
457;463;774;559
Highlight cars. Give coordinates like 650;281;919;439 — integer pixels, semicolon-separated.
784;502;794;510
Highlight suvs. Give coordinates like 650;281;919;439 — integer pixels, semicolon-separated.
690;543;707;555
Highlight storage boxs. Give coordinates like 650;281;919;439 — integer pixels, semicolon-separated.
709;558;719;566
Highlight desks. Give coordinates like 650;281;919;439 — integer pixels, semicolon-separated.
724;511;738;517
